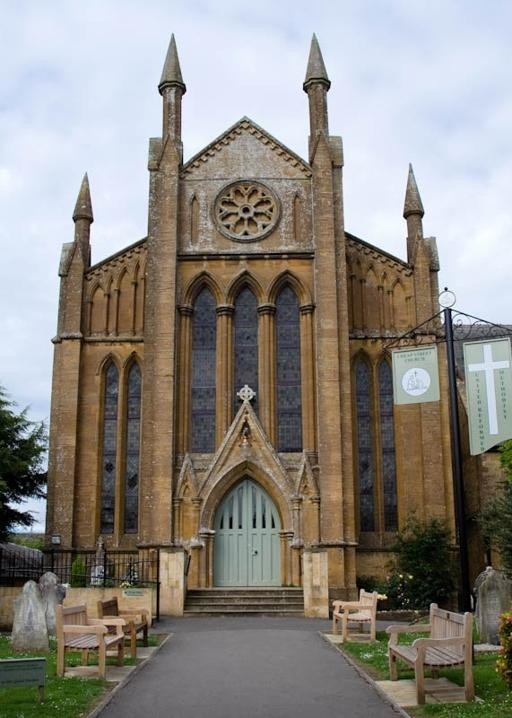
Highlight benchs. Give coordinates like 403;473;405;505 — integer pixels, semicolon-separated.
96;596;150;660
331;588;379;642
385;602;476;705
54;601;128;680
